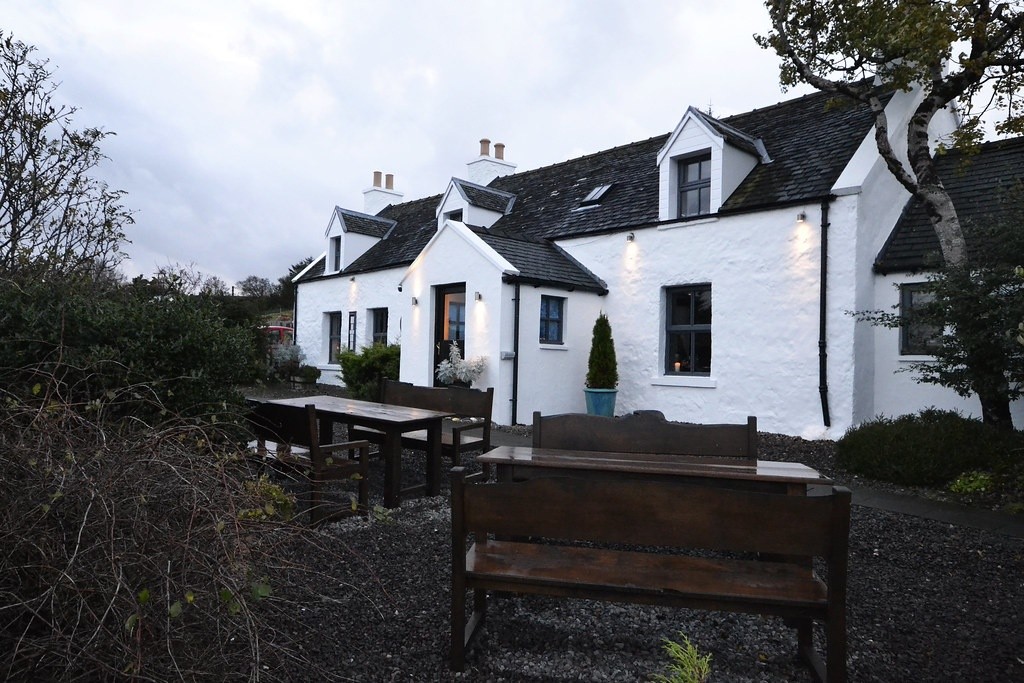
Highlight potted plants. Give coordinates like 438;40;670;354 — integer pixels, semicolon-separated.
583;314;620;418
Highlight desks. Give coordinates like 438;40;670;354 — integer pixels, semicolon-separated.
475;445;834;632
267;394;457;510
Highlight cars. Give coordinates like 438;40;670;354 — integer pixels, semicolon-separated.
256;324;294;347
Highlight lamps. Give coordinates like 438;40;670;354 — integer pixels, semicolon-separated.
627;232;634;243
797;213;806;222
412;296;418;306
474;292;482;300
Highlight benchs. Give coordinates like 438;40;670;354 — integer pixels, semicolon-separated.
212;379;852;683
